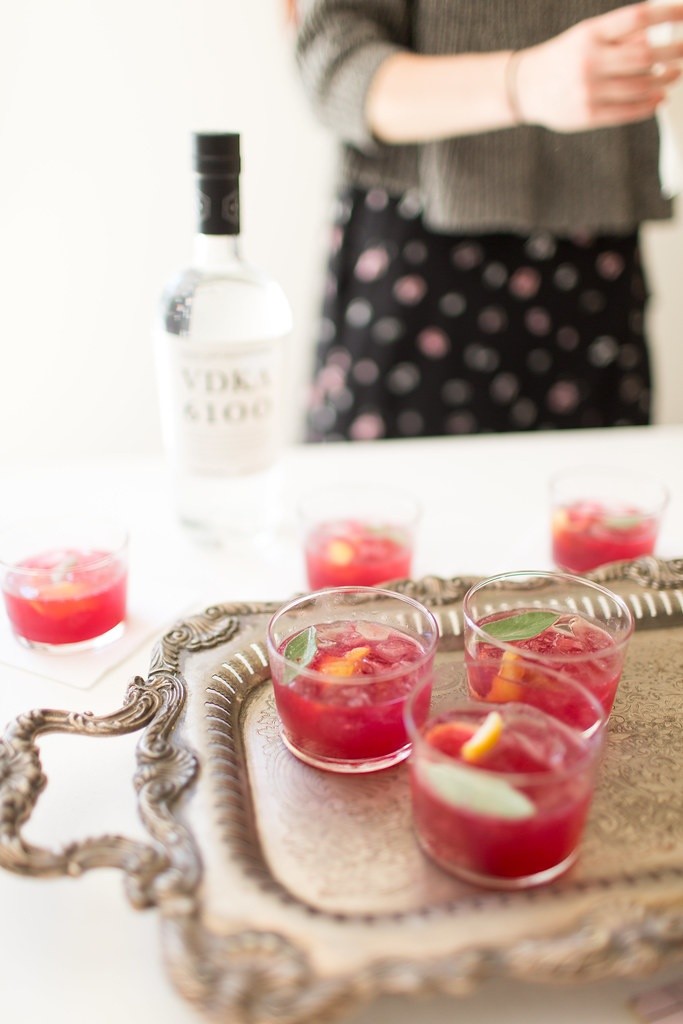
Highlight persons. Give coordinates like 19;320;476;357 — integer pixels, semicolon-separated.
276;1;683;443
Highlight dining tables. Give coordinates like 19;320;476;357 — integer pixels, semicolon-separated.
2;412;683;1024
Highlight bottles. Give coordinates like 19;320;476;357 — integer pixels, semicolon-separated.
156;127;279;592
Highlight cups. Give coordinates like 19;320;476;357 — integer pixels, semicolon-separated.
265;582;440;776
461;567;637;744
544;464;666;581
0;518;132;674
300;495;416;597
404;655;610;891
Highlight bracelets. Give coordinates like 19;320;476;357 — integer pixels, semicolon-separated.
501;46;531;129
